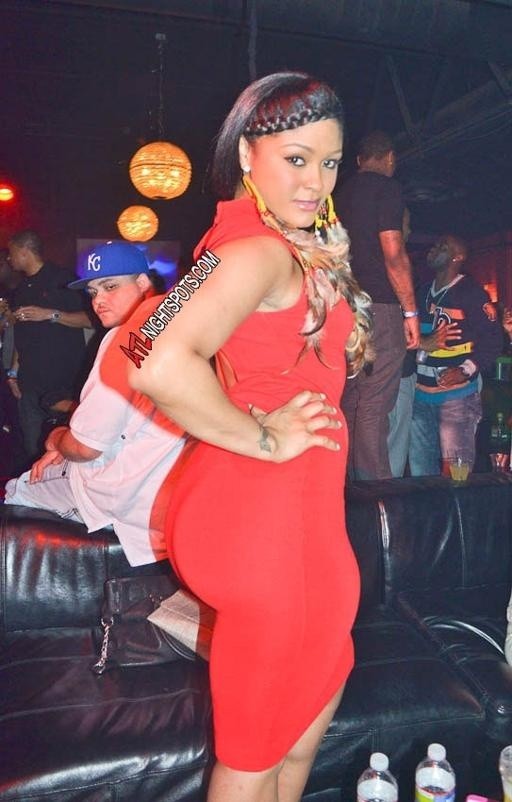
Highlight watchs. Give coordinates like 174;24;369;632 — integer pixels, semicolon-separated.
458;367;469;379
51;309;61;324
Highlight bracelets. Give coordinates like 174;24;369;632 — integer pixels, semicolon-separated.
402;311;420;318
6;370;18;379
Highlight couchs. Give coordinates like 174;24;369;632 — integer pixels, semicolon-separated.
1;473;511;802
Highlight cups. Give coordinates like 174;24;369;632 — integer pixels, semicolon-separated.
498;744;512;801
415;347;430;365
431;366;449;386
490;452;507;473
451;448;470;479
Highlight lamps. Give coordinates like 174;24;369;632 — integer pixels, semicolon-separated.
0;174;19;209
128;31;192;202
116;205;159;244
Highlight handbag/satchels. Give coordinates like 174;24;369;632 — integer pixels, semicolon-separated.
89;572;218;676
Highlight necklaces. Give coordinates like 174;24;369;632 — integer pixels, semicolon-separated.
425;281;451;314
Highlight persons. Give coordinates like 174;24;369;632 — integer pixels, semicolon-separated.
408;230;504;478
128;71;363;802
386;206;463;479
3;241;194;568
6;229;100;479
331;129;421;482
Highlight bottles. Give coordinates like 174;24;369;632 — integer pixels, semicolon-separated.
357;750;400;800
490;412;511;450
414;743;454;802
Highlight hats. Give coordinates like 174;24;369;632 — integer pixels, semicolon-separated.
67;238;152;291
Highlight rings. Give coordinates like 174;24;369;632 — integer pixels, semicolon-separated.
21;312;24;317
